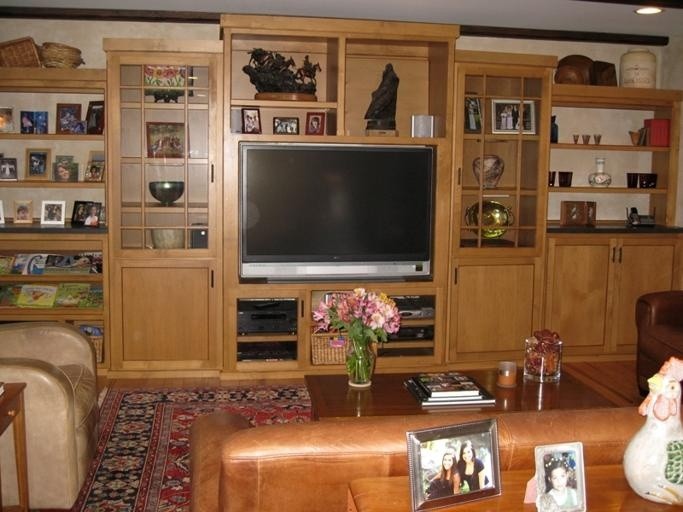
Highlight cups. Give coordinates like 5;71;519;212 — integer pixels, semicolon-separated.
626;173;638;187
572;134;601;145
557;171;573;187
638;173;657;188
549;171;555;187
497;361;517;387
524;337;561;384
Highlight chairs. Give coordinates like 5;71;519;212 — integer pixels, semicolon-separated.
0;318;101;511
633;289;682;402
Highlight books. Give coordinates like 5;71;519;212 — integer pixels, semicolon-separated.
0;253;104;308
403;371;497;409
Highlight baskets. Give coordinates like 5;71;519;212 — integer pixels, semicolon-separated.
42;42;82;68
311;325;377;366
0;36;42;67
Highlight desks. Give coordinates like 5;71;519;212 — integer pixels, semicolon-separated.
342;460;682;512
0;380;35;512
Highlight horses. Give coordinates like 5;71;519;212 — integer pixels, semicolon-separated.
248;45;322;89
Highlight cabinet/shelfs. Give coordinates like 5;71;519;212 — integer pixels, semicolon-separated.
547;82;682;234
101;37;223;260
219;13;461;144
224;282;446;383
443;255;542;371
540;233;683;364
447;49;561;262
106;255;225;381
0;66;111;383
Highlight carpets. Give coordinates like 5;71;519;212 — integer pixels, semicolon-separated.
35;370;317;511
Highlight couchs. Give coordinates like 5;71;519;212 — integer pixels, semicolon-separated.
185;399;682;512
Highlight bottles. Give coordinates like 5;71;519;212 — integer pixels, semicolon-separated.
550;116;558;142
588;157;612;187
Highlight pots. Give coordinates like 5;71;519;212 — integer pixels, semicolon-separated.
461;198;518;240
39;40;86;68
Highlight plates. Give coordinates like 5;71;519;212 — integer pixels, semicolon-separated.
557;55;595;85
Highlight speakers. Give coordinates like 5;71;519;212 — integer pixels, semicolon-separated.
412;115;434;138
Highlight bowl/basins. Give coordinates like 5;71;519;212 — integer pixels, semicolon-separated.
553;66;583;85
149;182;185;205
150;228;184;250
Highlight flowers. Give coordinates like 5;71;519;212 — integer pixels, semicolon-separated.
309;284;405;387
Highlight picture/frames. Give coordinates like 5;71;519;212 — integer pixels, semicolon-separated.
0;198;102;229
142;119;190;161
83;98;104;135
0;148;105;184
489;98;537;136
463;89;483;135
403;416;502;512
54;103;82;135
522;440;589;512
239;106;326;136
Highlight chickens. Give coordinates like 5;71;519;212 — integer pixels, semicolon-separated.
621;355;683;505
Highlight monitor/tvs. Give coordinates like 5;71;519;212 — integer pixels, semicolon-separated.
238;143;433;280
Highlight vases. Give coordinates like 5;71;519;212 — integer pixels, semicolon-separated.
585;156;612;189
343;332;377;389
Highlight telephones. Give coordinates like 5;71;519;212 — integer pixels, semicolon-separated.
626;207;655;225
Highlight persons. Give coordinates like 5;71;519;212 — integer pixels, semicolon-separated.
456;441;493;492
467;99;477;132
276;119;296;133
246;113;260;132
543;460;581;511
1;110;102;226
309;116;320;133
428;453;460;499
500;104;522;129
